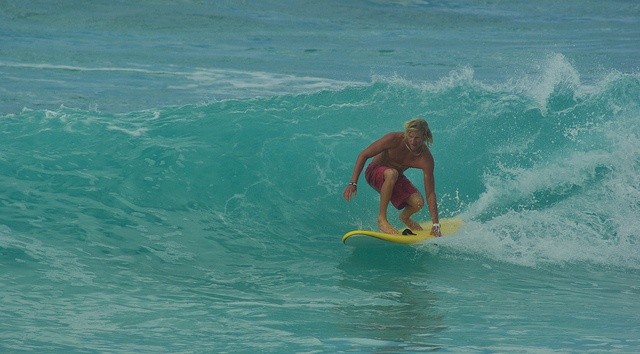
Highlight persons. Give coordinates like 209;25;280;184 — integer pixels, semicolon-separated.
343;119;441;238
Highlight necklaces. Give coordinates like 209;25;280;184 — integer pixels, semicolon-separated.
404;142;423;157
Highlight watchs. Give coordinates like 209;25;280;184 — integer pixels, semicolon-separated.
348;182;357;186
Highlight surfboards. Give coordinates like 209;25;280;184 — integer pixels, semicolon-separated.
343;216;463;247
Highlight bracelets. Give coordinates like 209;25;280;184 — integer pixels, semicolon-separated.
432;223;441;228
